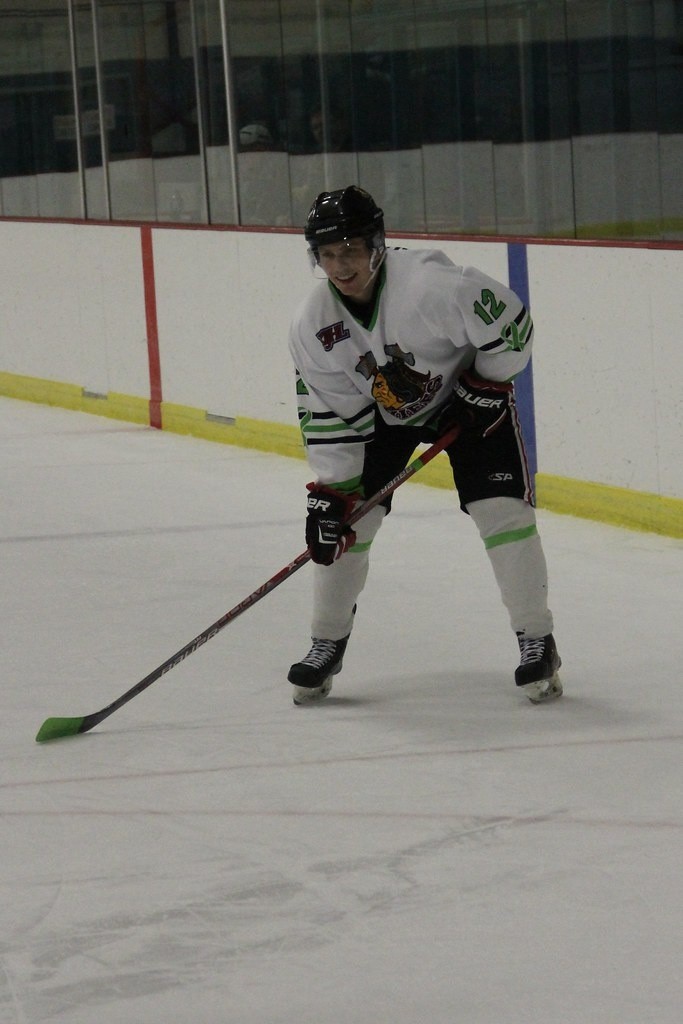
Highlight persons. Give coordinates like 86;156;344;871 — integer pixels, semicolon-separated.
287;186;563;707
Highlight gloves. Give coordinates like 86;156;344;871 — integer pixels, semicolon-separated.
305;482;356;566
437;354;514;457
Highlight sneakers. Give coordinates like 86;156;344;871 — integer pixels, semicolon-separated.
288;633;350;706
514;634;563;704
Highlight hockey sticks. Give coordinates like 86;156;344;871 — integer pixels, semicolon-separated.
34;426;460;743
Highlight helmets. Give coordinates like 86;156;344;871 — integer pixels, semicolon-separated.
304;184;385;264
